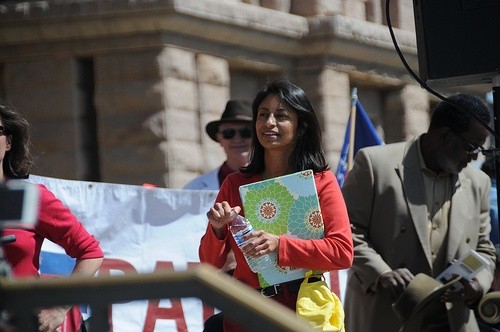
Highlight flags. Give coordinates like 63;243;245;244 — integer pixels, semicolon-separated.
336;95;386;190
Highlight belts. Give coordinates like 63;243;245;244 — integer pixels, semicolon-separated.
256;278;321;298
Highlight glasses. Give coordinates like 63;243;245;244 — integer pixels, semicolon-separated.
217;128;251;140
453;132;485;155
0;126;9;137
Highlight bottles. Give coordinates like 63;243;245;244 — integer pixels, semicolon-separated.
226;208;272;273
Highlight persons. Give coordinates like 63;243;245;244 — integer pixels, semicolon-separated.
342;94;496;332
0;105;104;332
207;99;256;275
198;80;354;332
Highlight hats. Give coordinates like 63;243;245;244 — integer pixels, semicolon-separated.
393;273;464;332
206;100;254;142
296;270;346;332
